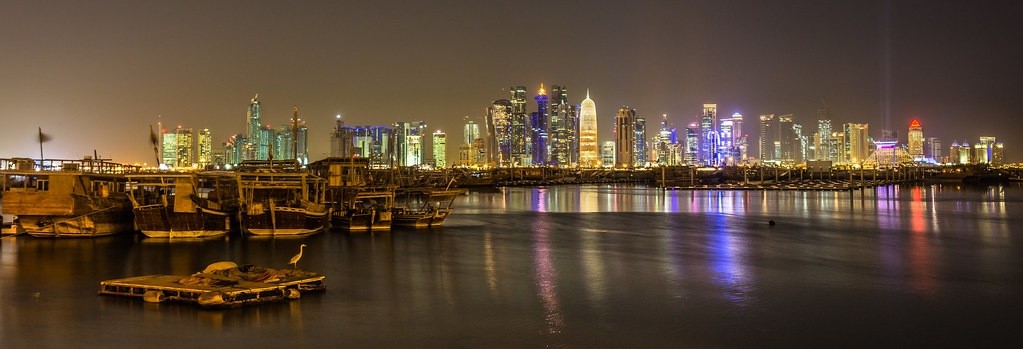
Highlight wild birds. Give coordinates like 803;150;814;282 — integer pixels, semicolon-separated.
288;244;307;269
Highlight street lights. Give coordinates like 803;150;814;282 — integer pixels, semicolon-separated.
157;122;163;165
203;128;209;168
175;125;183;169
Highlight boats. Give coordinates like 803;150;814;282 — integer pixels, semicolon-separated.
234;154;329;237
123;173;241;240
0;172;134;240
307;149;462;233
